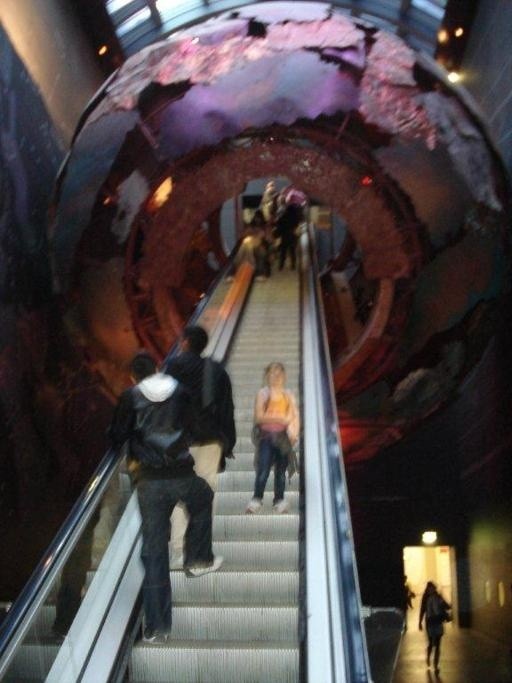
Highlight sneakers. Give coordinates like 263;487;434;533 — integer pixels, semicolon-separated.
184;555;224;578
141;632;169;646
272;501;289;515
246;499;263;514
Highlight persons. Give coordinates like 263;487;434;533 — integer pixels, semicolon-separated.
164;324;240;562
246;358;300;516
110;349;223;645
234;177;305;279
405;574;414;626
419;579;453;668
